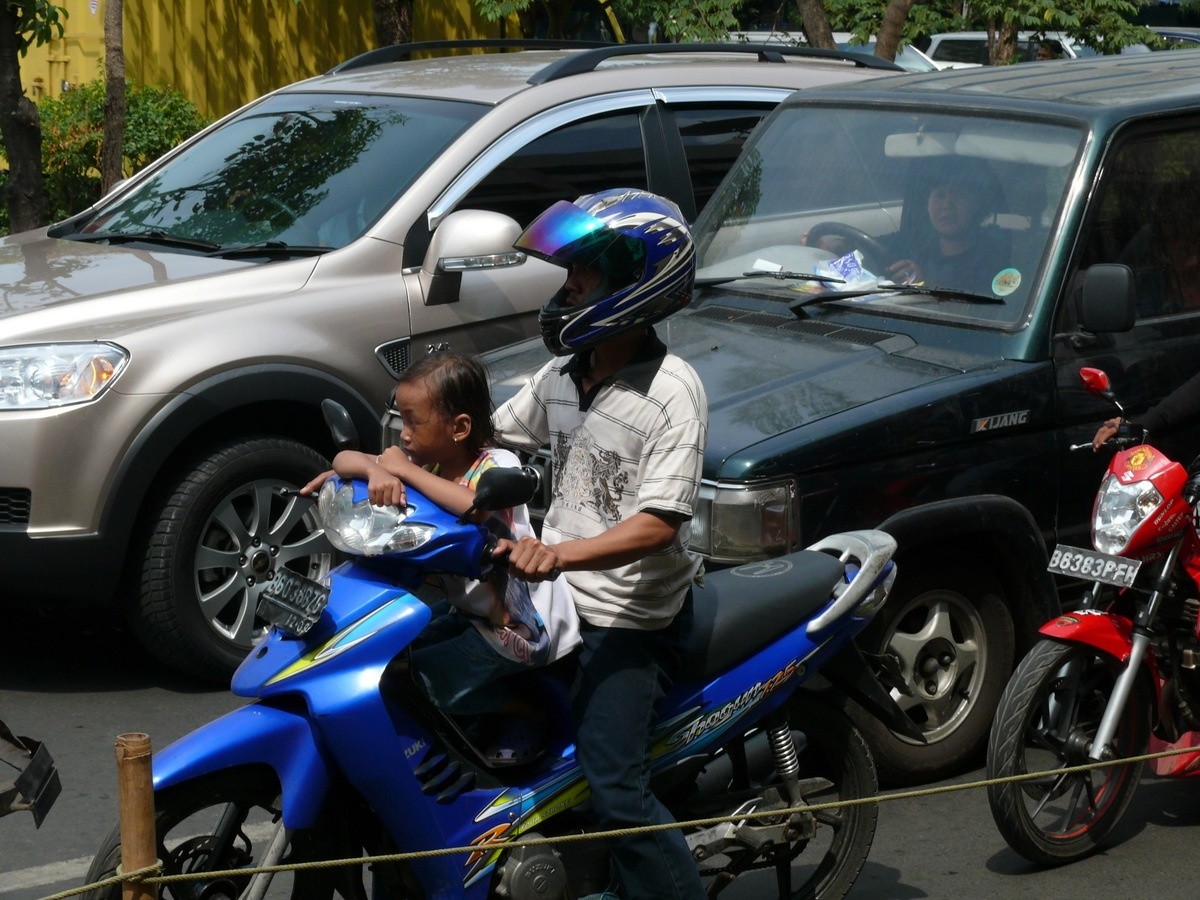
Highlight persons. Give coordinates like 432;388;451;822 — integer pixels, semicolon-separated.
801;159;1012;292
333;351;581;764
1035;39;1063;60
301;187;707;900
1094;373;1200;462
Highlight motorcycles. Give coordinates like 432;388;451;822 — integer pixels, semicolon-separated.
68;397;930;900
985;363;1200;869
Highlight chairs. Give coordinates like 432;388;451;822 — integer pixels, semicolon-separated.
1047;154;1170;304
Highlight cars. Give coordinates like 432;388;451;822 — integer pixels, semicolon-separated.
653;19;1200;68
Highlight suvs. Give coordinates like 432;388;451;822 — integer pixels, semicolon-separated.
0;34;955;694
365;43;1200;788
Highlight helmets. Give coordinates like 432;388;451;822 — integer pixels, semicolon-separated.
513;188;697;354
